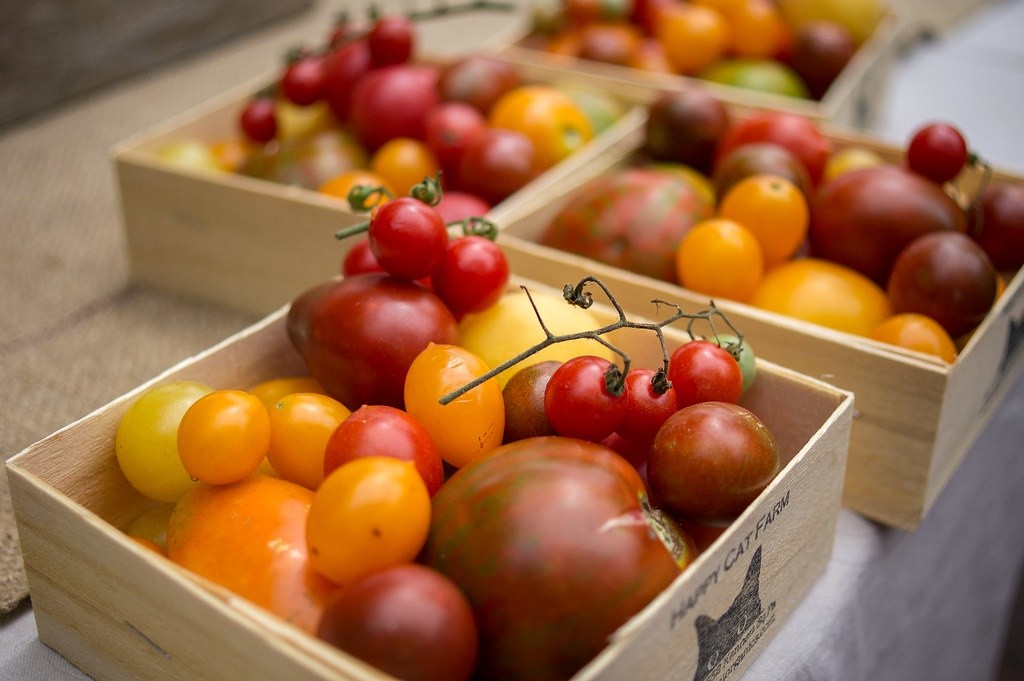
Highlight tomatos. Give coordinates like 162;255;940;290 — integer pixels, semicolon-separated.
530;88;1024;366
115;200;783;681
167;0;886;225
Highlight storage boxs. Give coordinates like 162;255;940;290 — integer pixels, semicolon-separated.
4;277;855;681
483;0;921;129
486;93;1023;532
110;46;640;312
